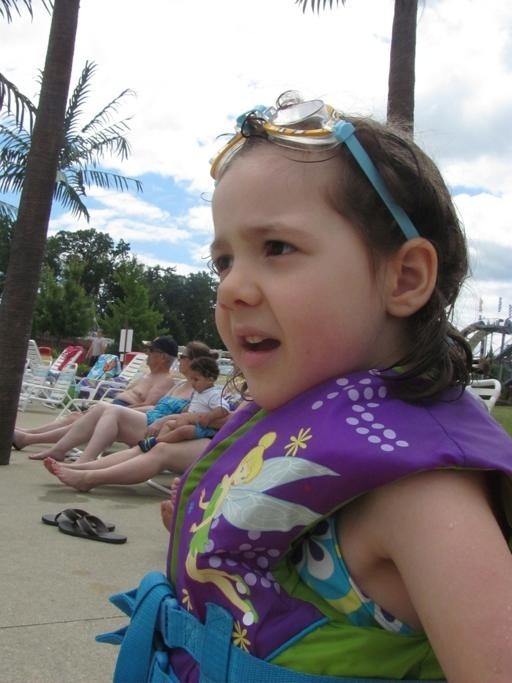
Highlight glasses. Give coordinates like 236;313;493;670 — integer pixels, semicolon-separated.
180;354;193;360
150;349;164;353
211;99;356;180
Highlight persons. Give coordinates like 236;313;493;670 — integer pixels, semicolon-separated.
85;328;108;368
94;91;512;683
13;335;252;494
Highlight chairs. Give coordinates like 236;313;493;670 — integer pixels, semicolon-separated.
17;339;501;495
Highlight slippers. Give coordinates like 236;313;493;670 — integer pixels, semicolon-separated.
42;508;127;544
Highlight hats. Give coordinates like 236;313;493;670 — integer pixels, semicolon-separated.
142;336;178;356
